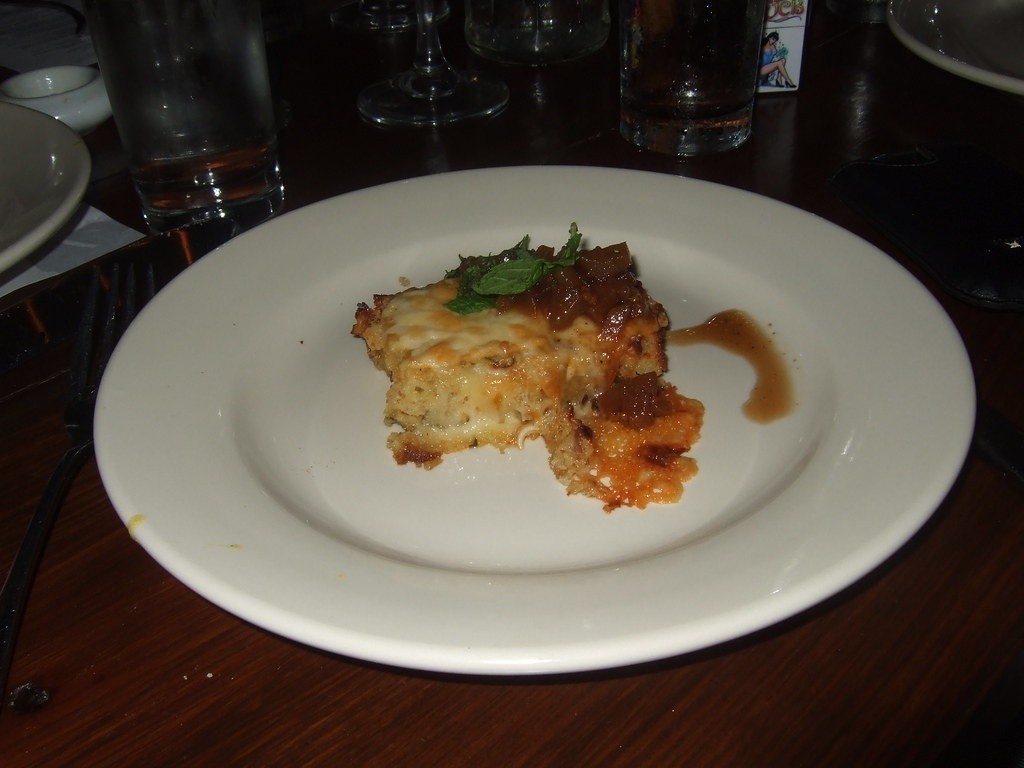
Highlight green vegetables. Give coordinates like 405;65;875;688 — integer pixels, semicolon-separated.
443;219;583;316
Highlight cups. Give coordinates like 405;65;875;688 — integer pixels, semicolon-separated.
463;0;612;66
618;0;764;156
82;0;286;236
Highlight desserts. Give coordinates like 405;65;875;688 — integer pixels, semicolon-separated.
350;239;671;469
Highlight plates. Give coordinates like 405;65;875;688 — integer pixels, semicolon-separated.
886;0;1024;96
1;101;92;275
92;166;978;672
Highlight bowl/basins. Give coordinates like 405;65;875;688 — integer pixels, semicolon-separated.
0;65;112;136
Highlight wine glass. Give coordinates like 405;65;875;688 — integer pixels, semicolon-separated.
356;0;511;132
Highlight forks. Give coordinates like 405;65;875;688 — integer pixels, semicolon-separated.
0;264;157;708
